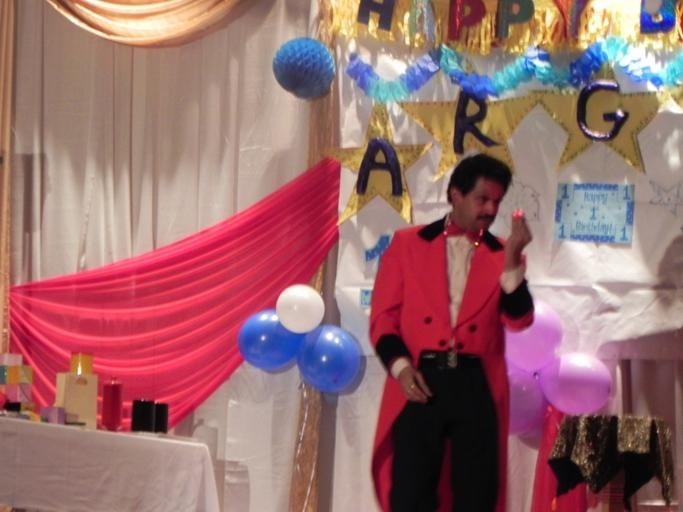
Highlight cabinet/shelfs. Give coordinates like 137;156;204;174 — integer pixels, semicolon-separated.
100;374;123;432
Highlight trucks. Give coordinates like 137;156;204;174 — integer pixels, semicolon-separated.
295;324;363;395
502;296;563;372
276;283;326;334
540;355;613;416
238;308;303;372
500;361;545;439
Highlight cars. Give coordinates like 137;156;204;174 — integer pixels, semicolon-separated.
69;352;93;375
131;399;156;434
154;403;168;435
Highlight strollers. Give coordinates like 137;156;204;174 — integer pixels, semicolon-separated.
442;224;481;248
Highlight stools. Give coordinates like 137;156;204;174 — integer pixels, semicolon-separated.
419;349;482;371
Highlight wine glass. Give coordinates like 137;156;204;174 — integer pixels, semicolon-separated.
410;383;416;389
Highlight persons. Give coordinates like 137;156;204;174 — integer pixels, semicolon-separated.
367;152;537;511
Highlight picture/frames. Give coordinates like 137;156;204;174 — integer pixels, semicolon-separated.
1;416;216;512
